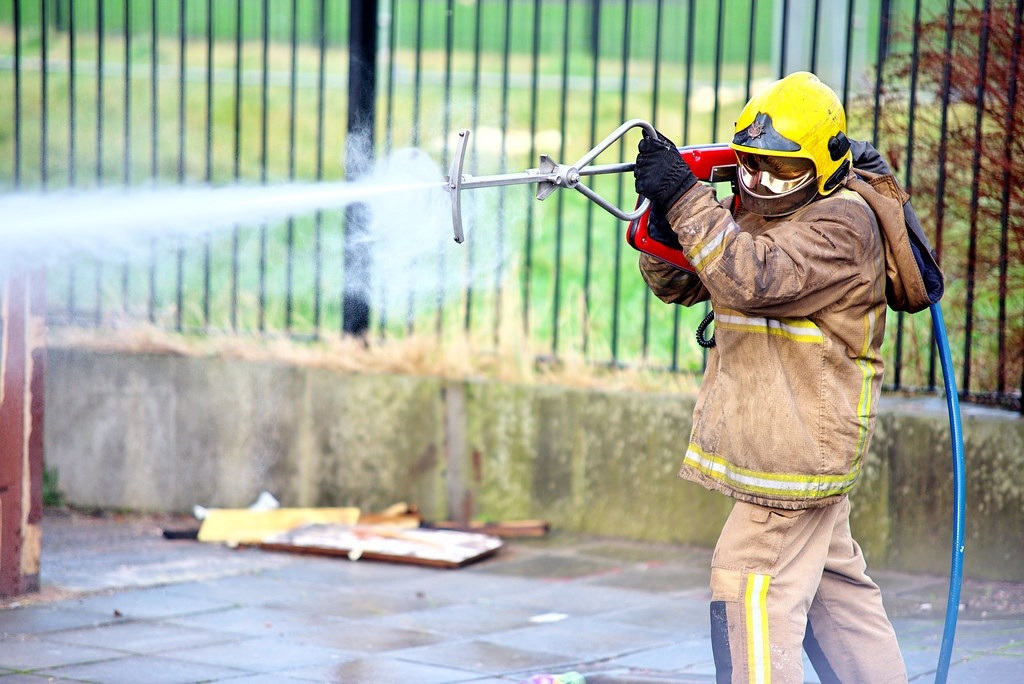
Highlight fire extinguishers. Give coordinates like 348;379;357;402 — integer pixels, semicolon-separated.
440;118;742;276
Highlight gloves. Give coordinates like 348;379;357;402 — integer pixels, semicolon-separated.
647;207;683;252
633;128;697;215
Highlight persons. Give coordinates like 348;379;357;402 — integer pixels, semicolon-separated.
634;71;945;684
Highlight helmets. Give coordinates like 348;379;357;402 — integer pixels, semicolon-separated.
729;70;853;217
